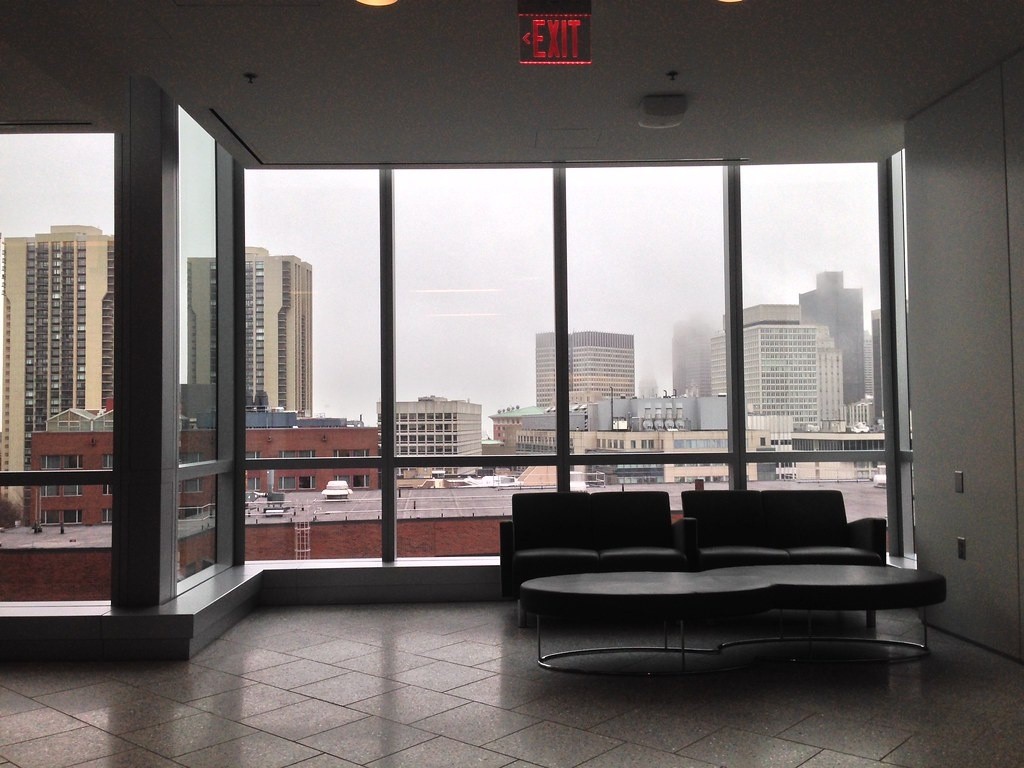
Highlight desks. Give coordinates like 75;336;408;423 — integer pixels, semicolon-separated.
522;564;946;674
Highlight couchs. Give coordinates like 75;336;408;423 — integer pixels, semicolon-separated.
499;490;887;631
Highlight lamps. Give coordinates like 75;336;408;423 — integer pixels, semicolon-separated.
631;90;689;131
